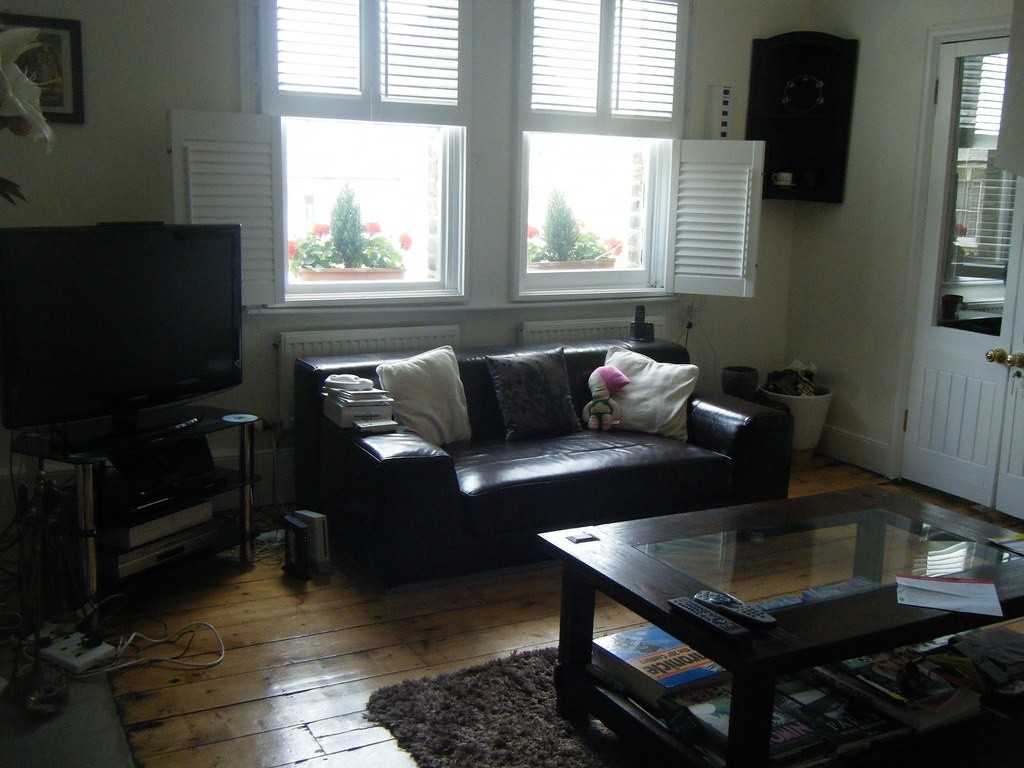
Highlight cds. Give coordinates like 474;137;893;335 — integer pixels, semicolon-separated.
222;413;259;422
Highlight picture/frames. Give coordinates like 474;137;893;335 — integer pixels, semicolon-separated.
0;12;86;125
722;366;760;399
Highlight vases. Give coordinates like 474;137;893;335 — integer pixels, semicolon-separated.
528;260;617;270
300;268;408;281
759;383;833;450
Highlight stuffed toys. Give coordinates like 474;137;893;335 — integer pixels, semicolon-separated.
584;366;630;430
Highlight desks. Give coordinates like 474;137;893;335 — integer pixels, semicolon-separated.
537;486;1024;768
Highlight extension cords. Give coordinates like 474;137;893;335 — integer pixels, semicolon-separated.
31;632;116;675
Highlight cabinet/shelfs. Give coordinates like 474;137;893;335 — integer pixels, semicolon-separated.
12;404;260;630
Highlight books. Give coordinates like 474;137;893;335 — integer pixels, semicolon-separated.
322;387;394;406
592;626;1024;768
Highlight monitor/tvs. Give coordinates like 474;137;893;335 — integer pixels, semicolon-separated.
0;222;245;447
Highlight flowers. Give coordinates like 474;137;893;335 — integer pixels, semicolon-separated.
527;190;625;264
950;225;975;262
288;184;414;277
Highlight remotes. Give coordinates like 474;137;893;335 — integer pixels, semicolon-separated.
694;590;777;630
667;596;749;640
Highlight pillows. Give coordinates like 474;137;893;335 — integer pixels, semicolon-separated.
484;346;583;441
604;346;699;443
376;345;472;445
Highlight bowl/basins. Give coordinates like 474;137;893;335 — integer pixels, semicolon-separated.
721;366;759;398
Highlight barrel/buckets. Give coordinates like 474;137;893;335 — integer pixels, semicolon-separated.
758;382;833;466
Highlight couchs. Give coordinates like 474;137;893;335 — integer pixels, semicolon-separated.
938;312;1002;336
293;338;795;587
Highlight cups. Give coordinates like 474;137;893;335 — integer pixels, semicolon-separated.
772;172;793;186
942;294;962;319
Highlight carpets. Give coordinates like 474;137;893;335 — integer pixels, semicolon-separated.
364;649;633;768
0;651;137;768
